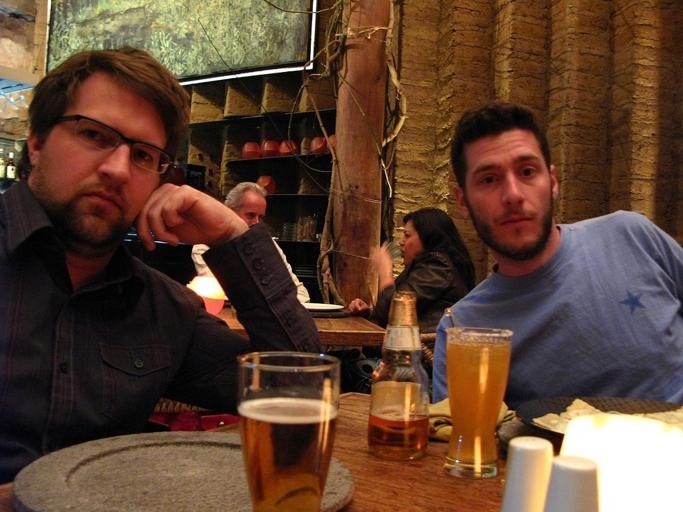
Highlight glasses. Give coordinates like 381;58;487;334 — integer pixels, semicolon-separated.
59;112;172;177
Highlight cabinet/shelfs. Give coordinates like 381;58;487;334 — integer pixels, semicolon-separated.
164;65;393;322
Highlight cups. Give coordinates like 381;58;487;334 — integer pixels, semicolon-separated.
441;321;516;480
235;348;341;511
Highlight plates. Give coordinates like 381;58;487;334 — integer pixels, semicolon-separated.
510;396;682;441
301;302;343;311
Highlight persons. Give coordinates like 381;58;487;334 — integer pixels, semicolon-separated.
323;206;476;394
432;103;683;408
0;46;327;483
190;182;312;313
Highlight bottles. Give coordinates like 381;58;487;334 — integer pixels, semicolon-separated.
272;214;318;244
5;152;16;181
0;147;5;180
367;287;432;467
498;436;552;512
545;454;601;512
